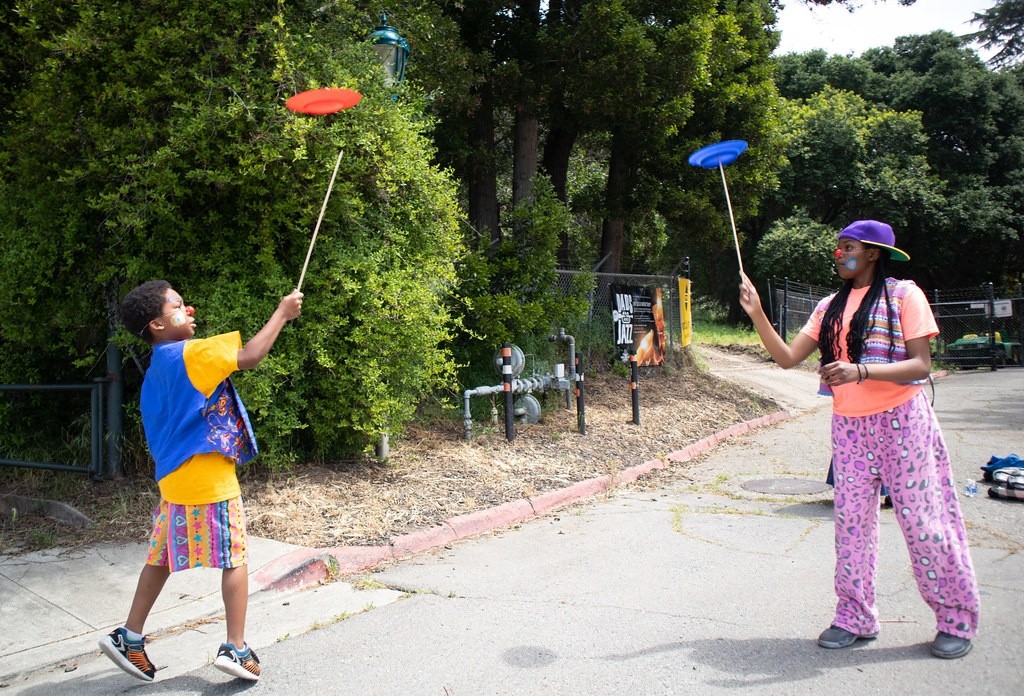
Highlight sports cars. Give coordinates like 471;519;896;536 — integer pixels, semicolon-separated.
945;331;1022;364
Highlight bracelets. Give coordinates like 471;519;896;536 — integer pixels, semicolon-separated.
856;363;868;385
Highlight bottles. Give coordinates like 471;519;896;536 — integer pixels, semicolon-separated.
964;479;977;497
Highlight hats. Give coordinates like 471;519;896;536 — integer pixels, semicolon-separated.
839;219;910;264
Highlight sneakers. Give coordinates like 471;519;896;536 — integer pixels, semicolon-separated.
215;641;262;682
99;627;157;682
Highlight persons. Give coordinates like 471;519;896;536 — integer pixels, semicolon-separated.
98;280;305;682
826;458;893;507
739;220;981;659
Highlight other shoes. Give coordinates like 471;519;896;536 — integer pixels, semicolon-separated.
931;630;971;659
816;624;858;648
885;495;892;508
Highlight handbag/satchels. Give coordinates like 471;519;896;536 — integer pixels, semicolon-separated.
989;466;1024;500
